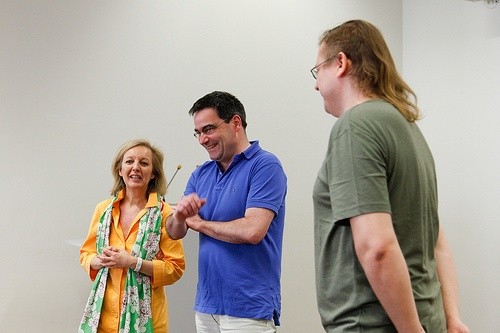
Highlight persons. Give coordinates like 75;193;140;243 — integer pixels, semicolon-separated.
165;90;287;333
310;19;470;333
78;139;185;333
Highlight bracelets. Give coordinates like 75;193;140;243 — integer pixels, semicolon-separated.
134;258;142;273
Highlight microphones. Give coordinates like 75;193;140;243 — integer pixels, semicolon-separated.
166;164;181;189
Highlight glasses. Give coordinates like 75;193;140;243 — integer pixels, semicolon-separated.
310;51;352;79
193;116;232;140
